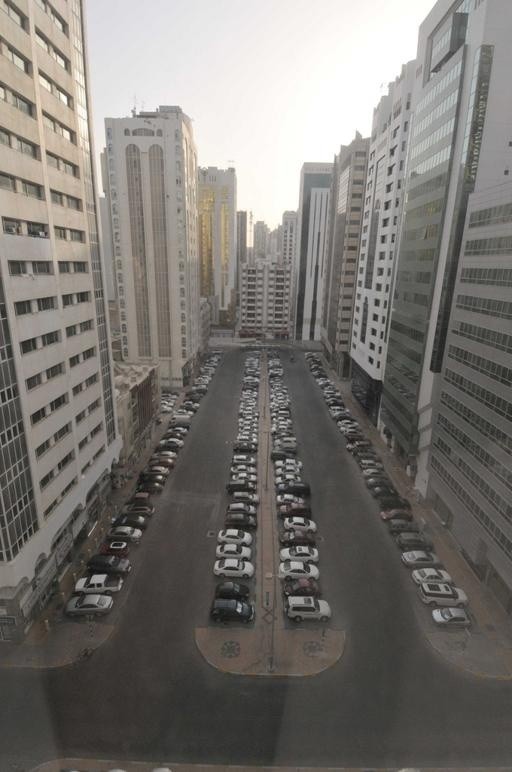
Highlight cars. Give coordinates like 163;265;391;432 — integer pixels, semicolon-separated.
211;350;332;624
64;492;156;615
323;386;434;551
135;358;217;495
401;550;471;628
